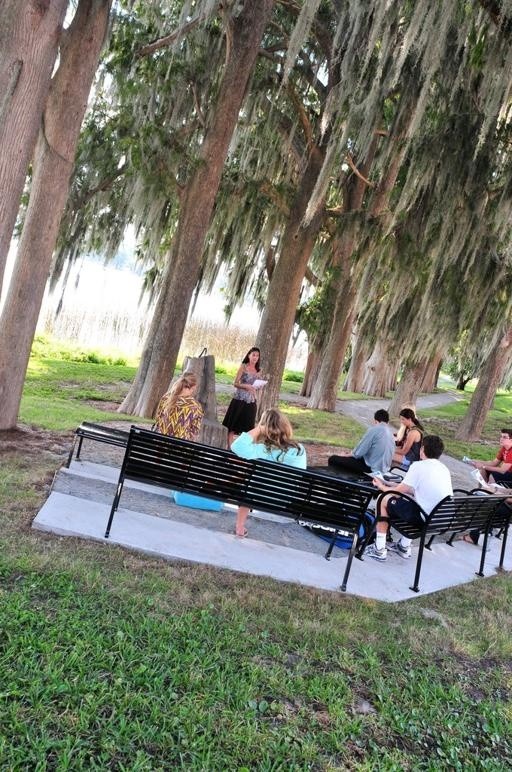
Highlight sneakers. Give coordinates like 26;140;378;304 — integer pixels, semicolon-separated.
359;543;387;562
386;540;411;558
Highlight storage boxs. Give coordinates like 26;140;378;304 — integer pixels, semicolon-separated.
172;488;225;511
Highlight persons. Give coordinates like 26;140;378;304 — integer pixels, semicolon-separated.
230;408;307;538
151;372;204;478
222;347;262;451
392;409;424;465
473;429;512;490
359;435;456;561
457;481;512;543
328;409;396;481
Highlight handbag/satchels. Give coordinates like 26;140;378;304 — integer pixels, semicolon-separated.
173;490;223;512
300;507;375;550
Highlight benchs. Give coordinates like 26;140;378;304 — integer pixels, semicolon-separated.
356;489;512;592
104;424;382;591
307;462;405;489
66;421;130;469
